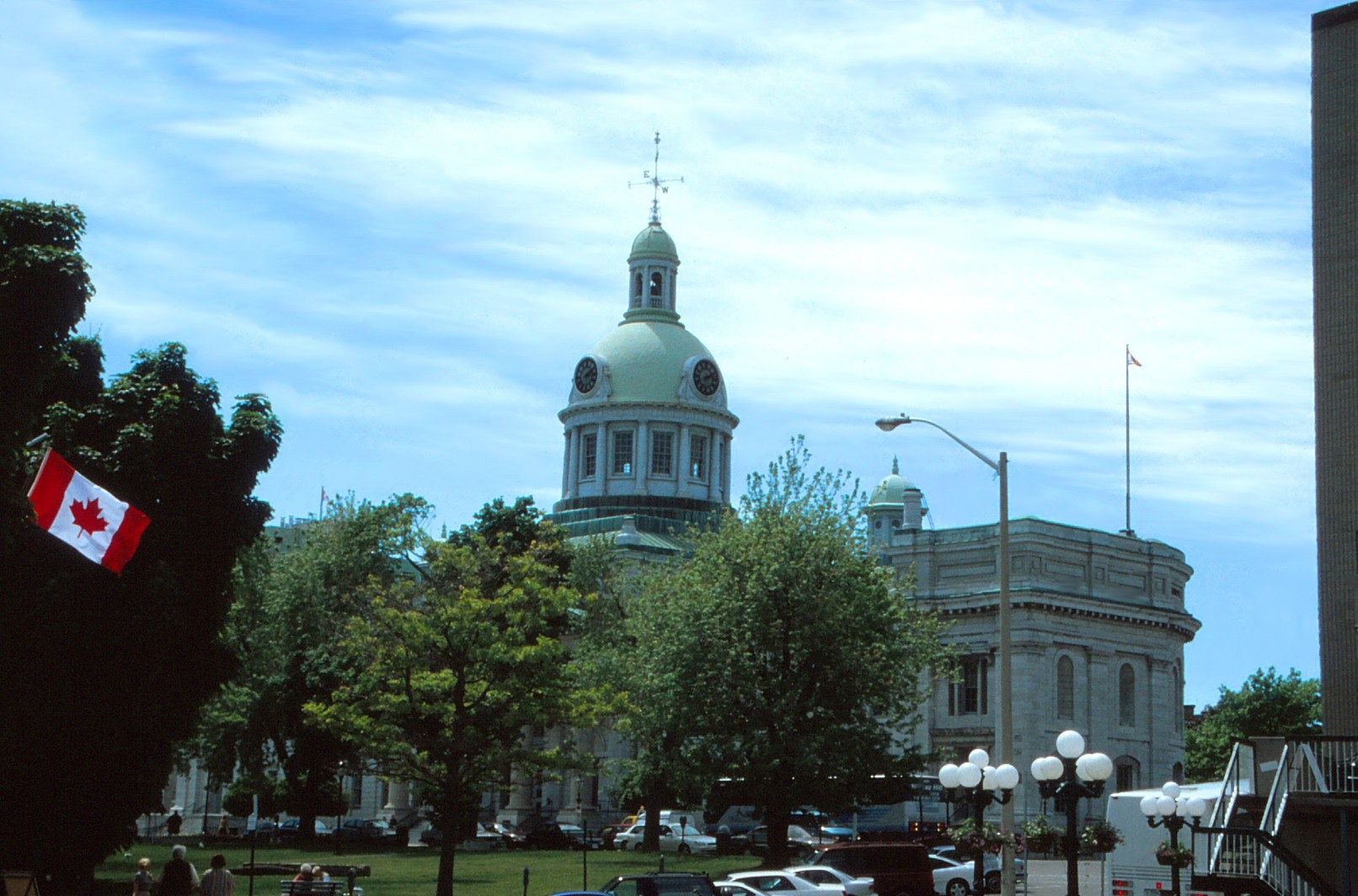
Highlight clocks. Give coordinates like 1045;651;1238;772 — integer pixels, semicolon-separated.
692;358;720;396
574;358;598;394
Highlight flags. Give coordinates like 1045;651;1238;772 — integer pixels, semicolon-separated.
26;448;151;578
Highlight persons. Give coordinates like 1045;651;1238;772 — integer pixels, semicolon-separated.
312;866;330;890
157;846;200;896
132;858;155;896
167;811;181;836
293;863;313;891
200;854;236;896
218;815;228;836
390;815;397;826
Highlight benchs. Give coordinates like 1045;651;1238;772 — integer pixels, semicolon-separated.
281;880;344;896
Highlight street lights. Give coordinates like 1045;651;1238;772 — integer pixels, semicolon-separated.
939;749;1020;896
1141;781;1206;896
1031;732;1112;895
335;761;350;856
575;775;586;827
875;418;1015;896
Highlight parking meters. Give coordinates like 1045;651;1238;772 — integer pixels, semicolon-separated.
679;815;686;856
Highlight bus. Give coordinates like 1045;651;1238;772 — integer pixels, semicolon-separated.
1107;778;1250;893
702;775;954;840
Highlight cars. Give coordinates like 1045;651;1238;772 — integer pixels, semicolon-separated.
248;816;398;844
555;841;1024;896
600;808;860;857
418;821;604;852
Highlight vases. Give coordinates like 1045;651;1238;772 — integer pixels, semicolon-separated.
1027;837;1051;853
1157;854;1189;868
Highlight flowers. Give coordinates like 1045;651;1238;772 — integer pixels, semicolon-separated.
1172;843;1194;863
948;815;1056;853
1077;819;1123;856
1157;841;1173;859
